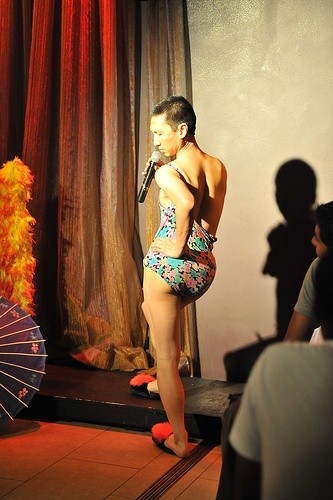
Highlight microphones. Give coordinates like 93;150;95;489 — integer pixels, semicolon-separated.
137;151;161;203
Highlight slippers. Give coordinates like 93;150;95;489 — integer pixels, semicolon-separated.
129;373;161;400
151;421;176;455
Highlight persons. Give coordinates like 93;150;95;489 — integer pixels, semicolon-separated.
215;200;333;500
130;96;227;458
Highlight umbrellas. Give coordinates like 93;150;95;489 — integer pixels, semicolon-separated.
0;298;48;428
